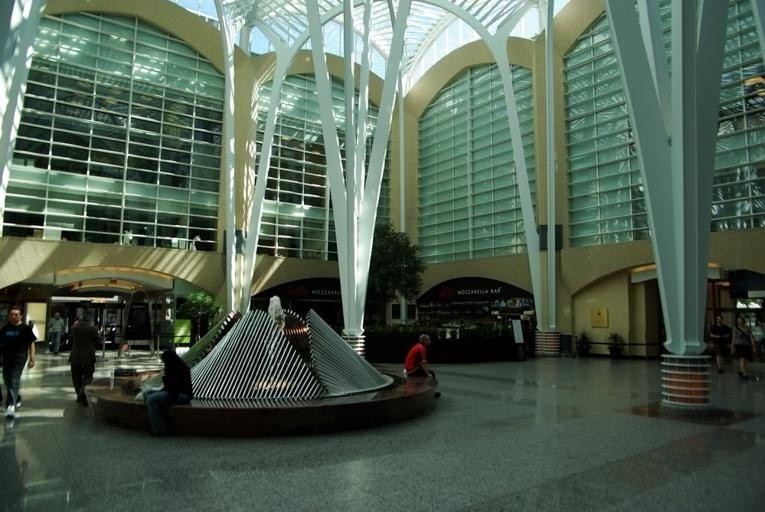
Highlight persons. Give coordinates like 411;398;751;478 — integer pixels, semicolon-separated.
46;311;65;353
66;307;102;408
731;316;757;380
143;350;193;437
709;314;732;374
404;334;442;398
0;305;37;419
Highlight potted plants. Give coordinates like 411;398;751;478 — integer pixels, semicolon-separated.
608;333;625;360
573;328;592;358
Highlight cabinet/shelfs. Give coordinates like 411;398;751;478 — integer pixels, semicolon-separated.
27;159;35;167
12;158;25;166
33;230;43;239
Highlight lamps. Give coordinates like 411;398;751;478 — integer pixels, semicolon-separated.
49;295;119;303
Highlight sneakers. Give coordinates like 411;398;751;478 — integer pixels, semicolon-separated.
15;395;22;407
76;394;88;406
5;405;14;418
434;392;441;398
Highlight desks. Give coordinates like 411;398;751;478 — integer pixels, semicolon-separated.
46;230;61;241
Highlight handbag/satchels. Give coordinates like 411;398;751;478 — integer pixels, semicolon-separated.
136;386;160;401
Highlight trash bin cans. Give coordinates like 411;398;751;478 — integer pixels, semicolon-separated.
560;335;572;358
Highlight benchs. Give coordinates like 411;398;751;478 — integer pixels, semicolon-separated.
118;340;155;358
